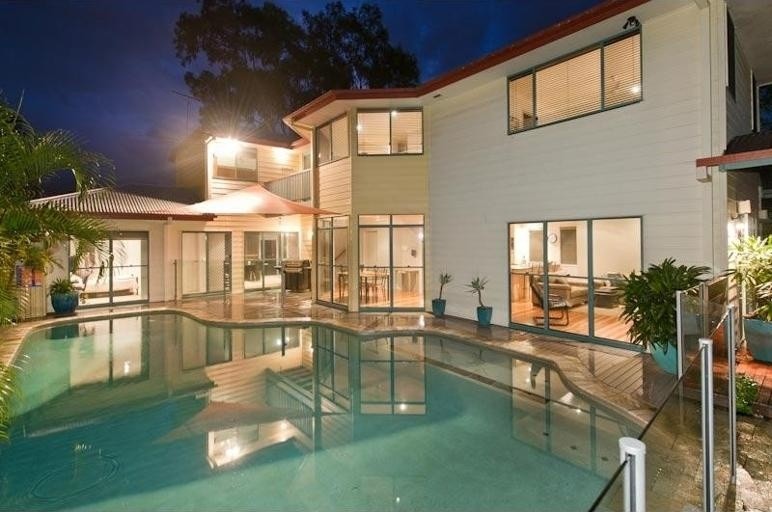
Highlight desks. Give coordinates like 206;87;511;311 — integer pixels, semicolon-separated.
337;270;390;303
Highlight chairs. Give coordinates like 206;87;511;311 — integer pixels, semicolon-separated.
337;263;387;304
529;275;570;327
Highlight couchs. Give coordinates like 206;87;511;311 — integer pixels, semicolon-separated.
531;272;603;306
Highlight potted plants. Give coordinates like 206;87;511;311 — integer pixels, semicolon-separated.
431;273;452;315
616;259;712;375
465;276;493;326
731;237;771;362
46;278;81;314
